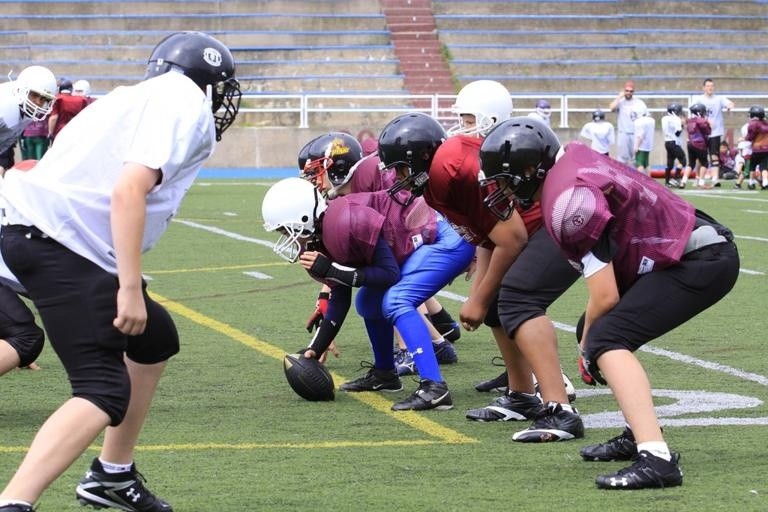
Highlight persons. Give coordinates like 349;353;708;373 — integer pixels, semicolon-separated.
295;132;462;379
1;29;243;511
478;115;741;492
449;79;580;406
373;112;587;444
1;63;99;377
296;134;463;337
261;176;477;413
531;77;768;193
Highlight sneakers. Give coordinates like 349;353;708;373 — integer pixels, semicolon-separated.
463;392;545;422
593;451;684;491
75;456;174;512
510;403;585;444
534;371;576;401
391;320;462;375
339;364;403;393
390;378;454;412
578;426;664;463
666;179;768;190
474;368;540;394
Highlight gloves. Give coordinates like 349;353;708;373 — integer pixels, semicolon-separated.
575;342;608;387
306;291;333;336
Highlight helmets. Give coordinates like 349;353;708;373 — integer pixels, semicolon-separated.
297;131;363;199
688;103;706;117
475;114;561;221
667;103;683;114
14;65;58;122
534;100;552;118
71;78;90;98
374;111;446;206
446;80;514;143
145;30;243;143
748;105;765;119
58;80;73;94
592;110;606;121
261;176;327;265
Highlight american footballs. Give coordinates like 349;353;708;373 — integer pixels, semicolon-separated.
284;354;334;401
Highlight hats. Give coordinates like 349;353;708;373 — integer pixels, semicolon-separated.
624;81;634;88
